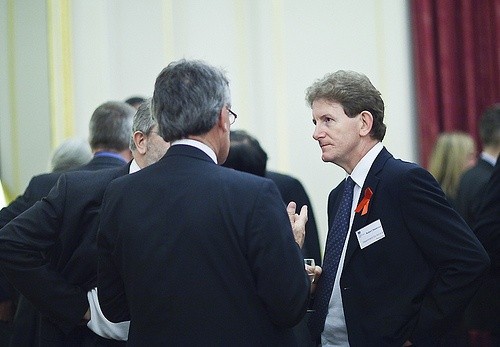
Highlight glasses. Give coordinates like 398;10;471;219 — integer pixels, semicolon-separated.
225;105;239;127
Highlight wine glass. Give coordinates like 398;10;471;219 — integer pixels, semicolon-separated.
304;258;316;312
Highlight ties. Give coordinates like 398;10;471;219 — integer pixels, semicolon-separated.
309;173;356;336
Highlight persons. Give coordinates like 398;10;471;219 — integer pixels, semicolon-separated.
427;102;500;263
96;62;311;347
0;96;323;347
306;71;492;347
